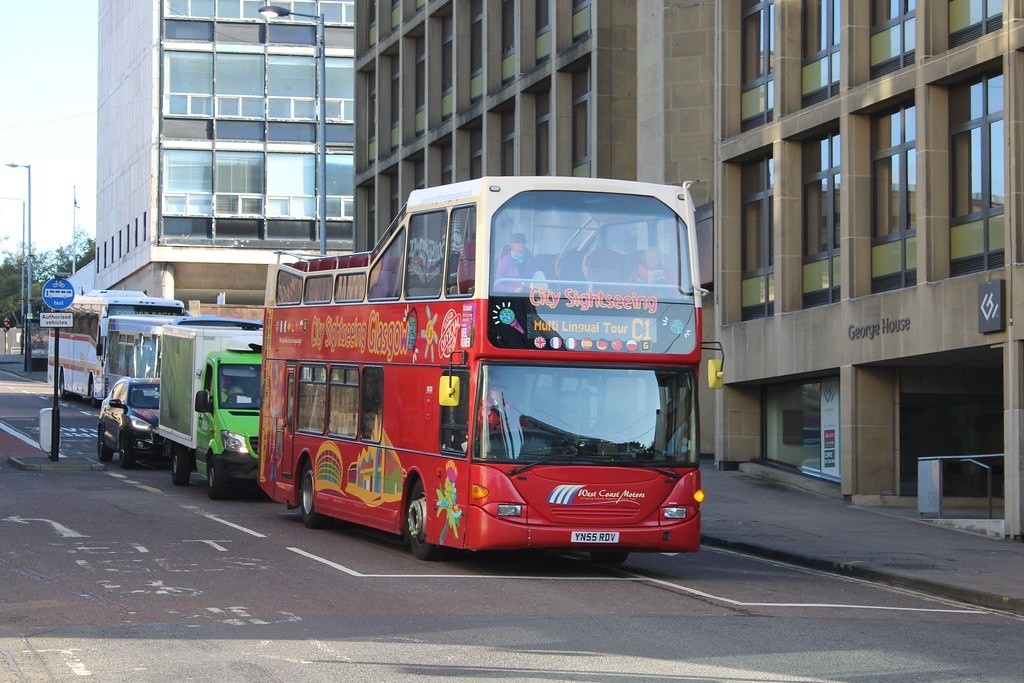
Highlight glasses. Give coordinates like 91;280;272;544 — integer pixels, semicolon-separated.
515;240;527;243
491;384;507;390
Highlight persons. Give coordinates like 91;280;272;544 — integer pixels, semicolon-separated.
363;374;381;433
496;233;539;293
219;375;244;402
625;247;676;286
476;376;527;439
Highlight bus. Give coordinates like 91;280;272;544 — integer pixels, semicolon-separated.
101;313;267;413
46;290;190;410
257;174;726;566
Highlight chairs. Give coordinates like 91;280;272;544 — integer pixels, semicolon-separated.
293;253;379;272
458;243;663;294
132;390;145;402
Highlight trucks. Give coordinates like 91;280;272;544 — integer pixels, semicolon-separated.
157;318;265;501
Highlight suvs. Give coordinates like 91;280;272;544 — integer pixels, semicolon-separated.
98;375;173;471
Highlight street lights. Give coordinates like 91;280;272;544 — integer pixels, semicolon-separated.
4;163;33;373
256;3;326;257
